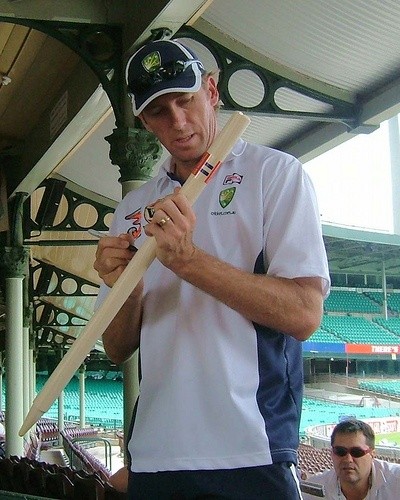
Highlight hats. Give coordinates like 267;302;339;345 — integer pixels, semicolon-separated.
123;38;206;116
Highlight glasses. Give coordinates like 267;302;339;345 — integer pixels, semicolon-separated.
333;446;372;458
127;58;203;97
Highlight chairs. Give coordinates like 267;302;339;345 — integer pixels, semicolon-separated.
0;368;128;500
301;281;400;344
297;372;400;476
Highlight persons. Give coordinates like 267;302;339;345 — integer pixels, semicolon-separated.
0;423;5;437
299;418;400;499
107;465;129;493
90;37;332;499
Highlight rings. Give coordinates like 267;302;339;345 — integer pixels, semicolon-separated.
158;215;172;227
